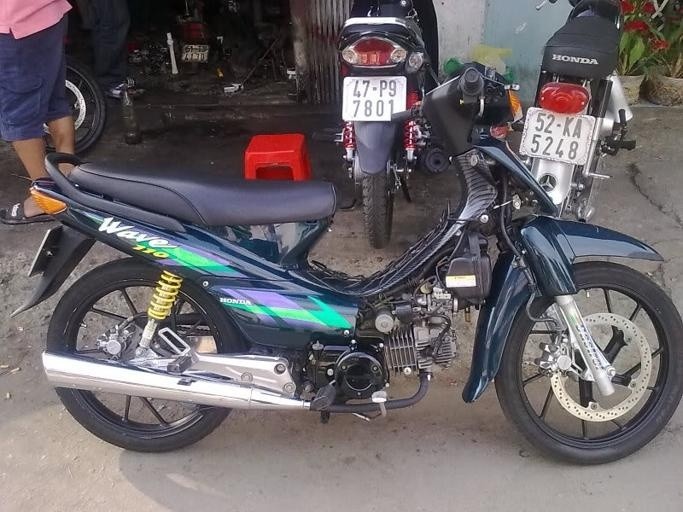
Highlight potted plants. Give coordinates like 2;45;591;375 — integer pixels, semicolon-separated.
647;22;682;108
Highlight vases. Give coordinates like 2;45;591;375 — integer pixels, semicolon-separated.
608;70;647;106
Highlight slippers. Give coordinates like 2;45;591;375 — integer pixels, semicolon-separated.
0;202;56;225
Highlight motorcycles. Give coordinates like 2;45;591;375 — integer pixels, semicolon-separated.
10;62;683;466
36;54;107;157
520;0;636;224
332;0;448;249
174;0;231;70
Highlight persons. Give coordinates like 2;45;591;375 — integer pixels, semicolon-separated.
64;2;142;98
0;2;76;227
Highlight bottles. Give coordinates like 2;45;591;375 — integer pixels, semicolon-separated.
120;85;143;145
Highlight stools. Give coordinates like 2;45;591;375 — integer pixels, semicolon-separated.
240;132;311;183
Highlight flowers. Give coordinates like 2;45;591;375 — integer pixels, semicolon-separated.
614;0;670;75
